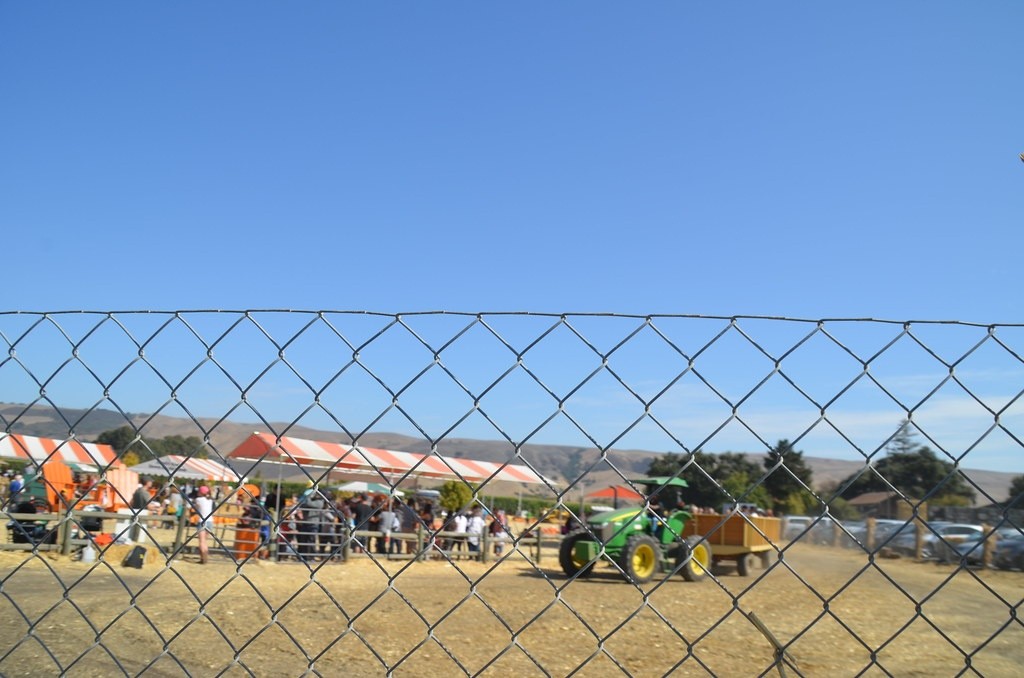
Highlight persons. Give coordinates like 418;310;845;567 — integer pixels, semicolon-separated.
647;492;665;538
130;476;158;545
161;486;213;564
238;483;510;562
7;474;25;510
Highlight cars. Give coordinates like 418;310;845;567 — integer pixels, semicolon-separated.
722;497;1024;573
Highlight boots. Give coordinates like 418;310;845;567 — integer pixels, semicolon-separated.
196;552;208;564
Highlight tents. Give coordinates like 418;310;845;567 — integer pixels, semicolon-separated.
220;434;562;556
330;481;404;497
0;432;123;515
128;455;248;483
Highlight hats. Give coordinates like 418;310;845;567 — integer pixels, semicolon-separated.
141;476;155;482
199;486;209;494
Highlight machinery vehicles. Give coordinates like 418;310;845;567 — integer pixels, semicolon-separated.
559;476;713;586
